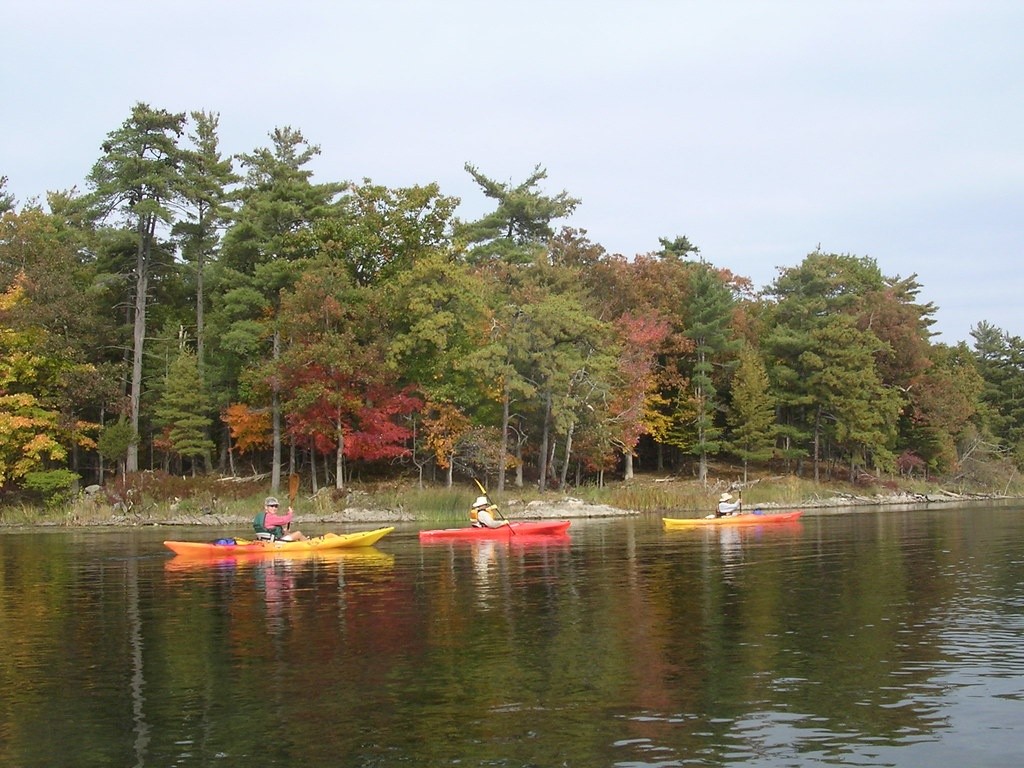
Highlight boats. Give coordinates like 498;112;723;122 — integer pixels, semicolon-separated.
420;519;571;538
164;546;395;574
662;511;804;526
163;526;394;556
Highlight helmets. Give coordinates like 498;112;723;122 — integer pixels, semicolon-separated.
266;498;280;506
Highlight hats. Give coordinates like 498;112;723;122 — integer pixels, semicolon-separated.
473;496;491;507
719;493;732;502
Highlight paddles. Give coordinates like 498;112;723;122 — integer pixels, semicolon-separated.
737;475;745;513
286;473;301;532
473;478;517;536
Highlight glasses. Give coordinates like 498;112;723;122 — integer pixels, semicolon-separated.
267;505;278;508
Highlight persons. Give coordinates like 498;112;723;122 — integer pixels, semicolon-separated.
253;497;308;543
470;497;509;528
716;493;742;518
